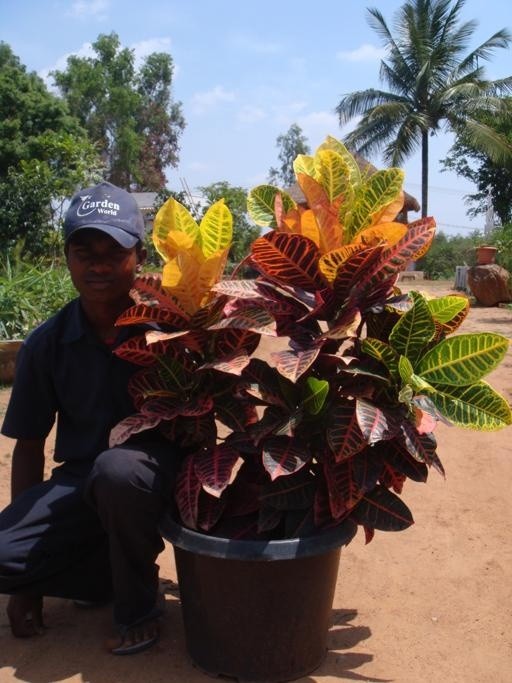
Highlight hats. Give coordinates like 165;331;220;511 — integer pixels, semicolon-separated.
63;182;144;248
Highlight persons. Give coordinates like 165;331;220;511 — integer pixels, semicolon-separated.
0;181;177;656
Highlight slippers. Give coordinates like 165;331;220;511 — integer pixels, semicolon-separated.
110;581;165;653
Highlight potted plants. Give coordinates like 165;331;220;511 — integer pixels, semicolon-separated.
105;132;512;682
0;251;81;386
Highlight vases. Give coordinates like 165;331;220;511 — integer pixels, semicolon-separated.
474;246;498;264
